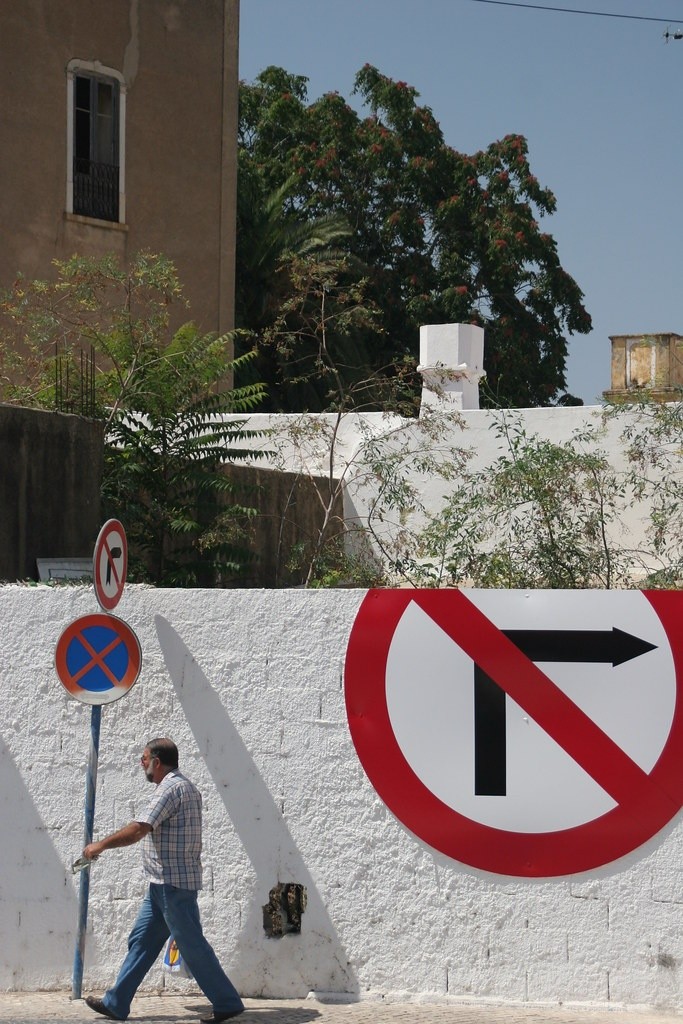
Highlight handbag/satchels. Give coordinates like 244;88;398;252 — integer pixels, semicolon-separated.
161;934;192;979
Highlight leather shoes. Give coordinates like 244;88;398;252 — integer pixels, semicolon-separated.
200;1009;244;1024
85;996;120;1019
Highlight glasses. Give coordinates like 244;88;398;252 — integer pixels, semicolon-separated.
140;756;155;765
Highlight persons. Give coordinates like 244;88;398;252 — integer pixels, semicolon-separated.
82;738;245;1024
